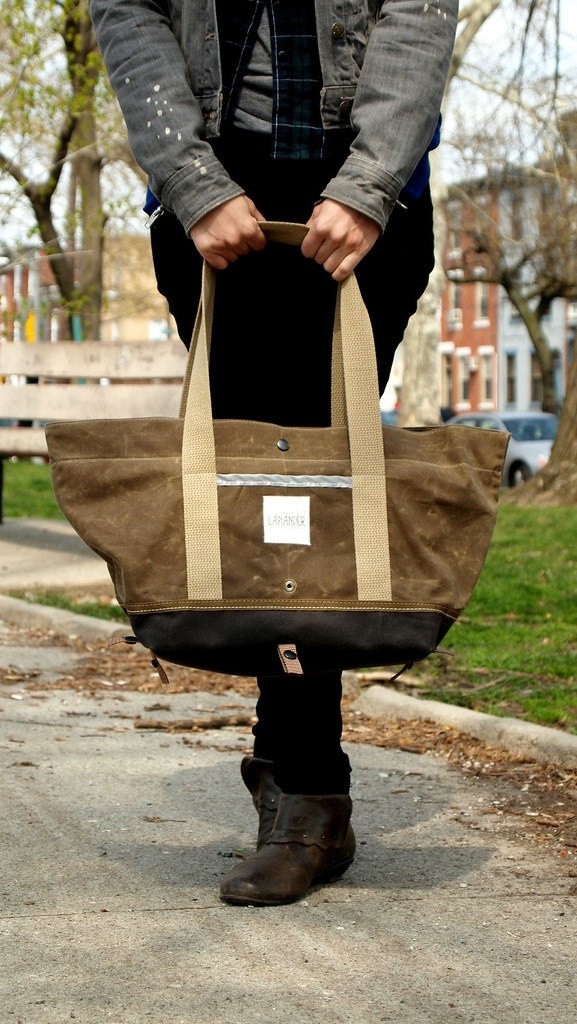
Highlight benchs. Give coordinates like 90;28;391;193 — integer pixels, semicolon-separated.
0;339;188;527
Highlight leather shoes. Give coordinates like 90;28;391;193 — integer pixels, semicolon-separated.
219;794;358;898
239;757;283;850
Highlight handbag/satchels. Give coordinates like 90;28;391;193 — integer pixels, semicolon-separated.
56;221;512;678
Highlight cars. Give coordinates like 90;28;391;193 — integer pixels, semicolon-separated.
444;412;562;490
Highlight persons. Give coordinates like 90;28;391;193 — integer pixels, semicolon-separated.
88;0;461;909
